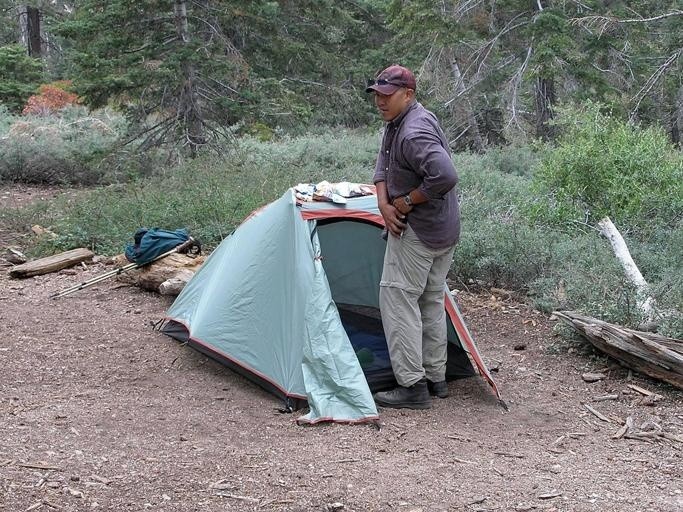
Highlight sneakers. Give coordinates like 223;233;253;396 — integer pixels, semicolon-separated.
374;378;449;410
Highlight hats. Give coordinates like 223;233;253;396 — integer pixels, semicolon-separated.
366;64;416;96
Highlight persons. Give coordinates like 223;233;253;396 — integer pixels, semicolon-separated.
365;64;461;410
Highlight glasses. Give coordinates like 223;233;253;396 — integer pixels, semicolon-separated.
367;78;400;87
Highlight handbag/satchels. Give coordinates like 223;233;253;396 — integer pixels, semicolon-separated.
125;229;201;266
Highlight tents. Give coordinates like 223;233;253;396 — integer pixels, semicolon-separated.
161;181;509;428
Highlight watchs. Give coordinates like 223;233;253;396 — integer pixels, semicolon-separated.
404;195;413;206
